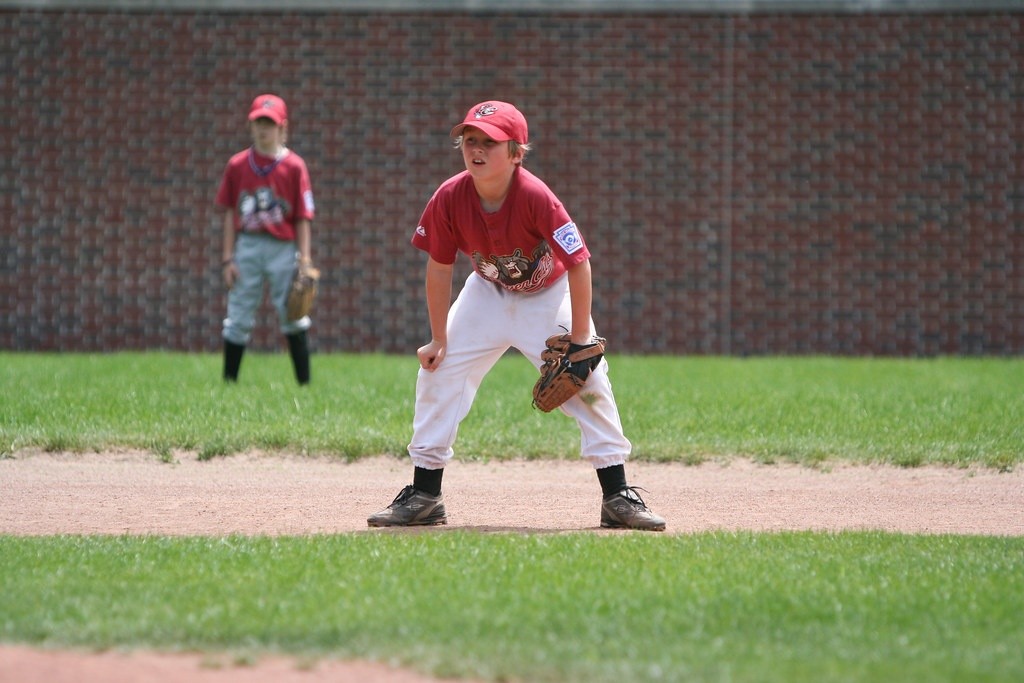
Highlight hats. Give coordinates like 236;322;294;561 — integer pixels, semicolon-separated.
450;101;528;145
249;94;287;125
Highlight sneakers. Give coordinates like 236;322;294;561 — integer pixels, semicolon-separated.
601;486;666;531
367;484;447;527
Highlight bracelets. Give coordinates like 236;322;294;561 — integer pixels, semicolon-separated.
221;259;230;270
248;145;284;176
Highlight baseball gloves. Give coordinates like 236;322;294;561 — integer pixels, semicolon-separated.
532;333;607;413
283;266;322;322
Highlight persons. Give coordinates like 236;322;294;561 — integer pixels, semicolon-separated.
366;100;667;532
214;94;316;386
